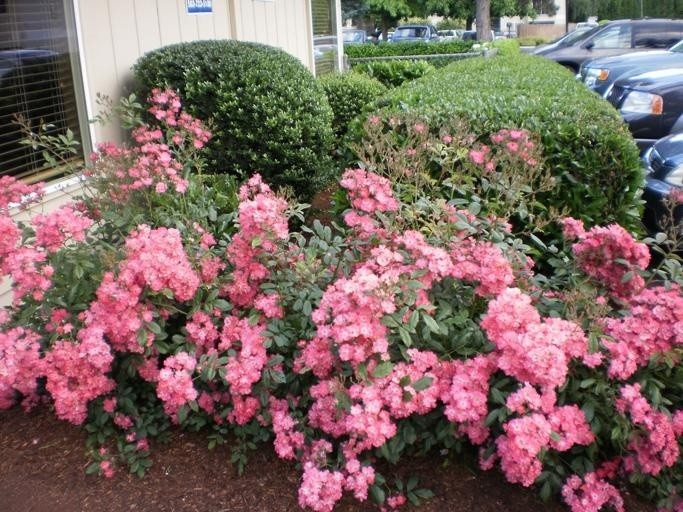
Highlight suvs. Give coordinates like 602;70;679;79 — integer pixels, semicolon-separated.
313;36;336;59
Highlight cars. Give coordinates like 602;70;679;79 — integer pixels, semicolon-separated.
529;19;683;252
0;50;61;114
343;24;508;48
